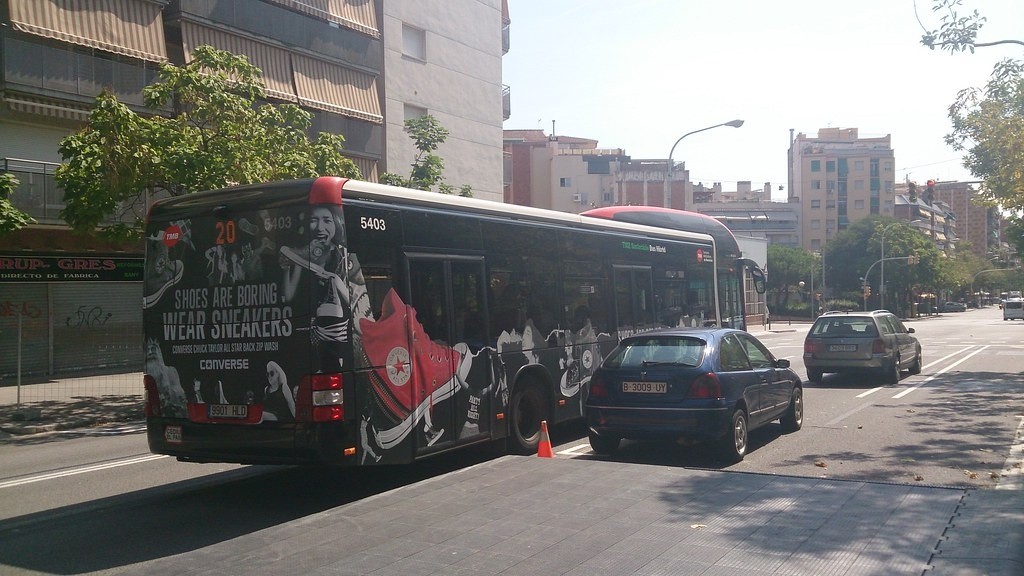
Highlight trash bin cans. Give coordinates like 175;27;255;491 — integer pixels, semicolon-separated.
909;303;919;318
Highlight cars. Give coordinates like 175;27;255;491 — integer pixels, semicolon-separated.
583;327;804;463
938;289;1024;321
802;307;923;385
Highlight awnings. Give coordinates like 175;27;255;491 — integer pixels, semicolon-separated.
3;0;383;124
340;155;378;183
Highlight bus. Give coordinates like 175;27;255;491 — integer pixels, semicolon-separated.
140;175;769;469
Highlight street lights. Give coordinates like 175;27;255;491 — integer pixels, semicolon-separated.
880;219;923;310
821;240;833;313
666;118;745;209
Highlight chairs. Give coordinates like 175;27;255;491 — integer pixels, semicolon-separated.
831;324;876;334
651;348;676;363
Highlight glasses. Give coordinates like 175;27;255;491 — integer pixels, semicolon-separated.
265;370;274;377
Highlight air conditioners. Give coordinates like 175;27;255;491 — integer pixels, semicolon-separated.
573;193;582;202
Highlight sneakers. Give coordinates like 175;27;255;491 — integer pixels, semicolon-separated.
375;455;383;464
423;424;444;447
140;259;185;309
357;287;473;450
560;317;604;398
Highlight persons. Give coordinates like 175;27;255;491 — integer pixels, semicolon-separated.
192;361;295;420
147;343;188;416
766;305;769;324
275;208;347;302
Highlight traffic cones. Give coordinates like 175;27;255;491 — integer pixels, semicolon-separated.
535;420;555;458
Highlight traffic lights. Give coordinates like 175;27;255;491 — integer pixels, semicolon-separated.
907;254;914;265
866;286;872;297
914;254;920;265
815;293;822;301
909;181;918;204
927;180;937;202
